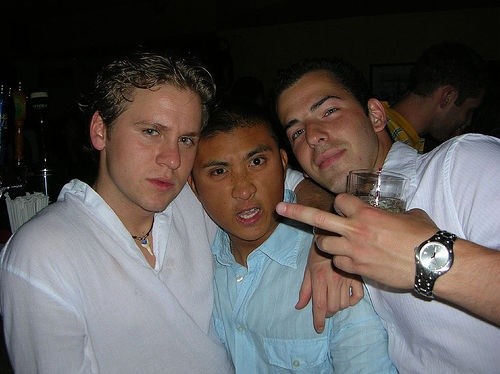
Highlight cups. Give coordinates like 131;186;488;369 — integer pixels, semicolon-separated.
346;168;411;214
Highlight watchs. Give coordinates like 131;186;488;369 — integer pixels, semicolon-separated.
411;230;456;299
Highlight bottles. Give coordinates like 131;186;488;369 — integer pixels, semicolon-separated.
0;79;28;168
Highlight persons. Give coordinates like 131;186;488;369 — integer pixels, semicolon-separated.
377;42;500;155
187;88;399;374
273;55;500;373
0;43;364;374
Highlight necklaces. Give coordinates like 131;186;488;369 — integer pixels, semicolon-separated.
131;217;154;256
